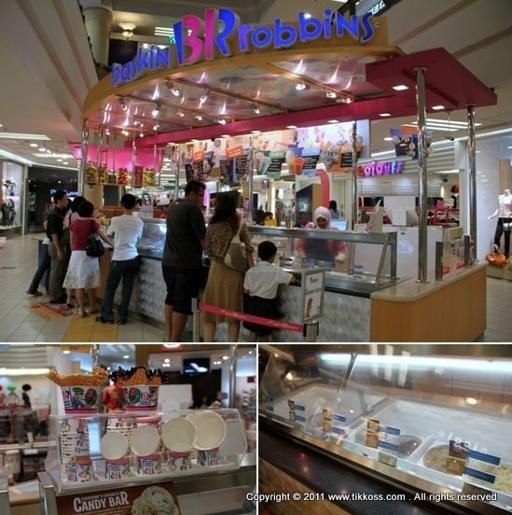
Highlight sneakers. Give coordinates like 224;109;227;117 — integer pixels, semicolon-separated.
23;288;128;326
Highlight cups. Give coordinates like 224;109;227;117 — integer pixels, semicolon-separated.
60;386;227;491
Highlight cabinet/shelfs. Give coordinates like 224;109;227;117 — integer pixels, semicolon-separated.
1;366;250;512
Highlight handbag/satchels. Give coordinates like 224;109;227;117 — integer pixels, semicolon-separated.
85;220;104;257
223;220;250;273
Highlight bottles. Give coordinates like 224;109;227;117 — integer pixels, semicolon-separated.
0;385;20;444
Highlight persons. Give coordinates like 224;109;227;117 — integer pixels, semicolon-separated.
26;191;144;325
297;200;345;268
162;180;295;342
488;189;512;259
